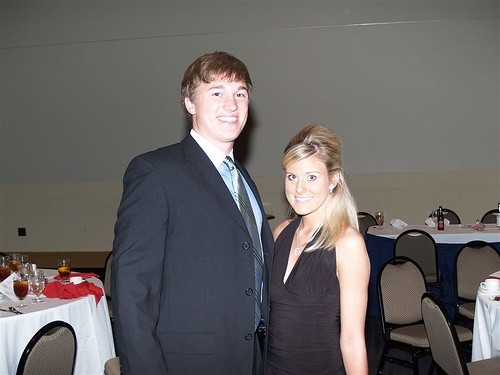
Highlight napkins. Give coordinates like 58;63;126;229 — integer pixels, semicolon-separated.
53;271;100;282
425;216;450;227
40;281;104;306
390;219;408;228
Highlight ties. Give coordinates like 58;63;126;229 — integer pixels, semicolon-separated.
223;155;263;331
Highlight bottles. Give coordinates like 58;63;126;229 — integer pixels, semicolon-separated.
437;205;444;230
496;203;500;227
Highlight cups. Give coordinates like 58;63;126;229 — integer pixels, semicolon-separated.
70;277;82;285
0;253;34;283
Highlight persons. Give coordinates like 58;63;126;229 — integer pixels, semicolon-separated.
108;51;274;375
266;124;370;375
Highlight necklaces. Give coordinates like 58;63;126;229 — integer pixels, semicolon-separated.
294;233;301;256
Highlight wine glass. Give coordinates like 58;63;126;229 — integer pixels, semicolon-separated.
57;256;71;285
375;211;385;230
30;272;45;304
432;211;437;229
13;277;29;309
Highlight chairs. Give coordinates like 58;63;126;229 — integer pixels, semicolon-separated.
357;212;378;239
16;321;78;375
425;208;461;224
480;209;500;224
376;229;500;375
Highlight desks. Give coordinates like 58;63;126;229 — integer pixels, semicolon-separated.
367;224;500;319
470;270;500;363
0;269;117;375
